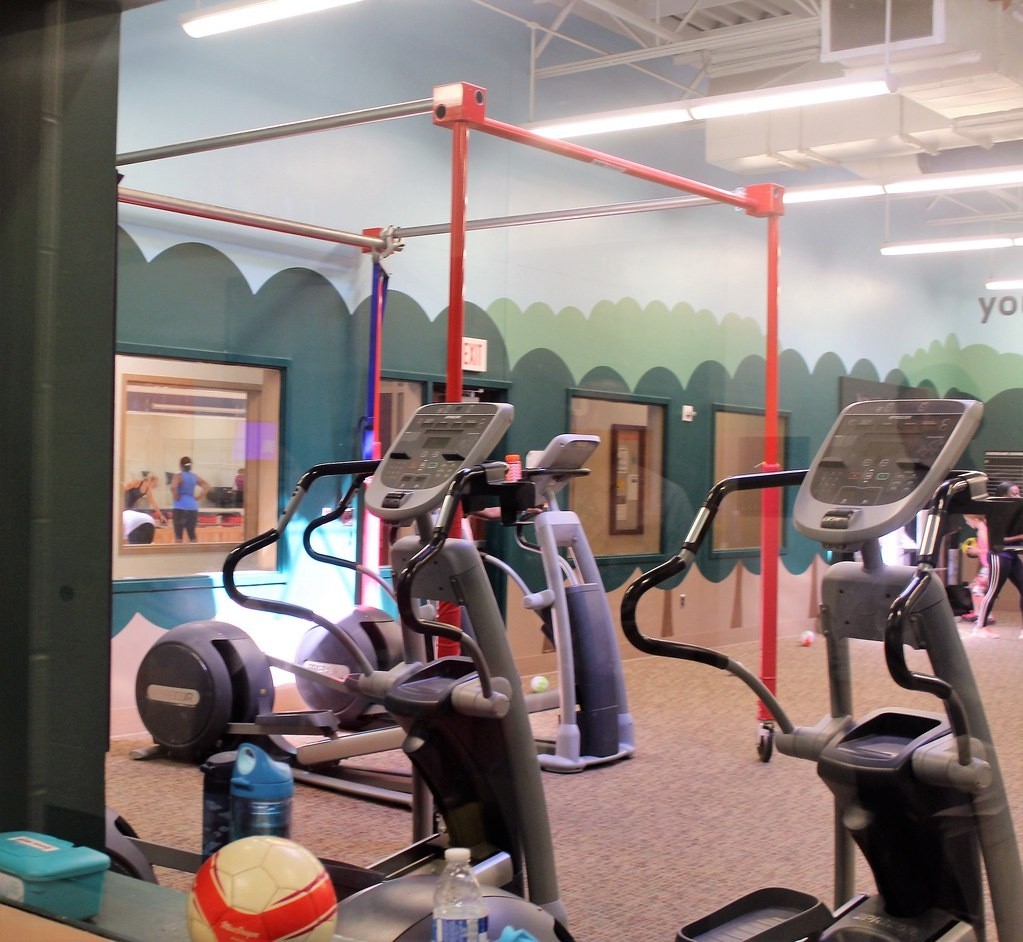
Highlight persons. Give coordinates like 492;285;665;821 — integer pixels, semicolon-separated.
172;456;211;542
962;513;995;624
124;472;168;523
233;468;244;509
123;511;155;544
974;481;1023;639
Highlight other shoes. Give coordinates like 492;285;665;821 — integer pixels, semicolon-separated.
973;628;998;639
986;613;997;624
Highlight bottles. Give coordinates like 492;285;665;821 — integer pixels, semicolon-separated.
431;848;489;942
505;455;522;481
200;751;236;866
230;743;293;845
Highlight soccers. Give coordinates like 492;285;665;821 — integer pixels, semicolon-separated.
185;833;340;942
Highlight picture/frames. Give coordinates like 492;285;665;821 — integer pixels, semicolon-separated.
609;424;645;535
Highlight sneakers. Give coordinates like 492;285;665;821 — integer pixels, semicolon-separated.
963;611;979;622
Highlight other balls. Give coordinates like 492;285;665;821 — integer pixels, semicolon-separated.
529;675;549;692
799;630;816;646
961;536;978;558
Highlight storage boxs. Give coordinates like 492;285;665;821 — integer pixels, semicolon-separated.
1;830;111;920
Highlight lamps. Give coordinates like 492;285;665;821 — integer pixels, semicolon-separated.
985;280;1022;290
178;0;363;41
518;69;898;141
781;163;1022;204
879;233;1023;257
